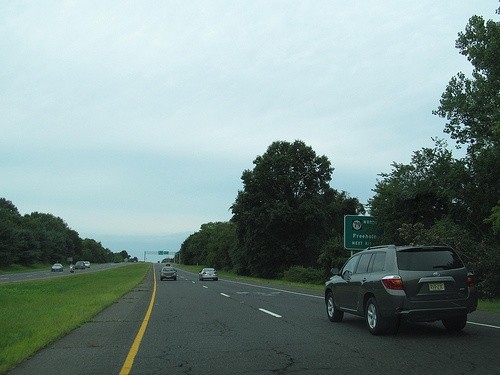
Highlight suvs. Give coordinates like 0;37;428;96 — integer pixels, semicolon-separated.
324;244;479;336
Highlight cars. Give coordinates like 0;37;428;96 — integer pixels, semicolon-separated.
198;267;219;281
159;266;178;280
51;263;64;272
75;261;86;270
84;261;90;268
